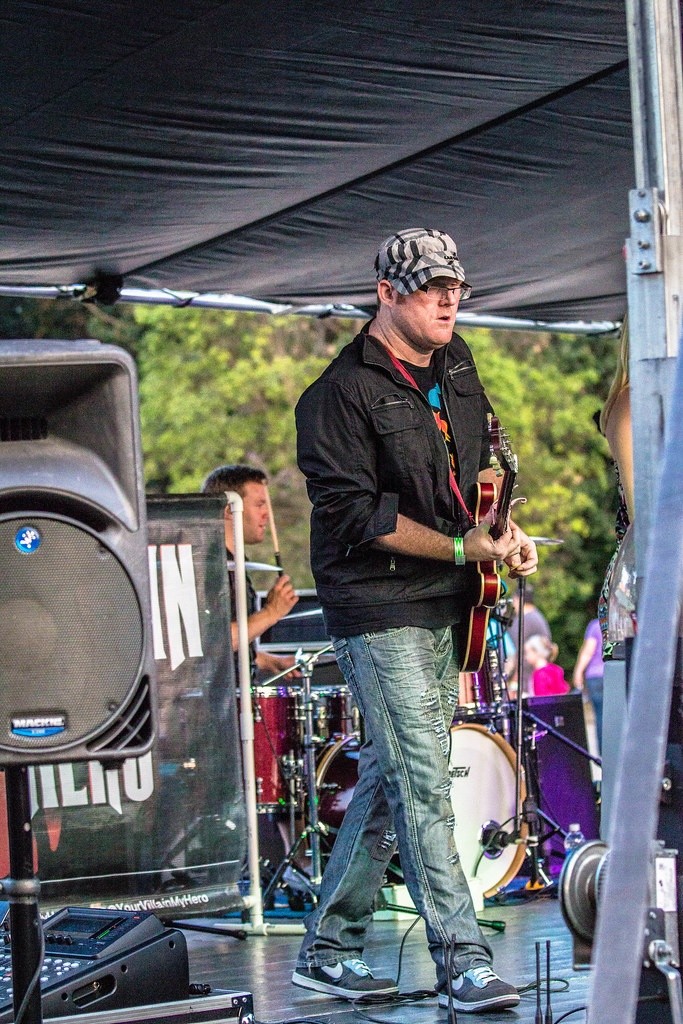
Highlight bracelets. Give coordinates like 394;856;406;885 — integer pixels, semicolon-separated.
454;536;466;566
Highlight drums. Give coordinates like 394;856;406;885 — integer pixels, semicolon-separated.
234;682;354;809
454;642;507;720
403;720;531;904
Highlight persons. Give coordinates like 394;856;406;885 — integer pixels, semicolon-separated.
203;465;323;892
572;618;604;757
486;583;553;699
525;634;570;695
291;226;540;1014
599;312;682;743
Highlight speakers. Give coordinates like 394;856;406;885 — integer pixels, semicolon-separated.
512;692;602;876
0;339;247;923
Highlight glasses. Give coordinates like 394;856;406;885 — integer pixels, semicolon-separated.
419;281;472;299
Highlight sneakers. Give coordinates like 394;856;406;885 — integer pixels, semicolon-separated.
291;950;399;1001
437;964;520;1015
285;863;330;893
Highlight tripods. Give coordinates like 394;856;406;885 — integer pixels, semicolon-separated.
260;644;409;907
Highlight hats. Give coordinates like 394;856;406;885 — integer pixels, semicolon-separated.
375;227;465;292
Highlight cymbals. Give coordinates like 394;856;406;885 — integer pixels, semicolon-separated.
225;560;284;575
530;534;566;550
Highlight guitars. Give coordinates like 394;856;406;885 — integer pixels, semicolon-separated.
453;413;503;672
